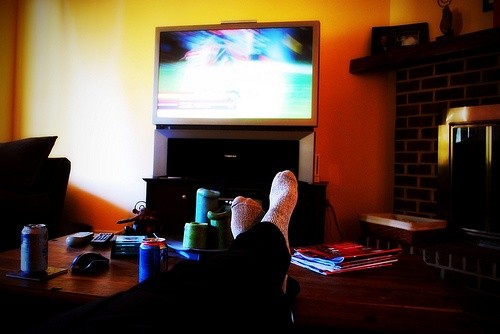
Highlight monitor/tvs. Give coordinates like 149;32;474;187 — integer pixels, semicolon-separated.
154;20;320;130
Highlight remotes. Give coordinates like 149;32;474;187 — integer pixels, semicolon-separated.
90;232;113;247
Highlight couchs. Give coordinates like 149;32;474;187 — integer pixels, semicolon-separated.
0;158;70;253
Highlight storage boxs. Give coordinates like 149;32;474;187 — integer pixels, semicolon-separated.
110;235;147;255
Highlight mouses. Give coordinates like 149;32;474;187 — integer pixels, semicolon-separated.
70;252;110;276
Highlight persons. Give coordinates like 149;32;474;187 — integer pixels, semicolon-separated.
2;169;299;333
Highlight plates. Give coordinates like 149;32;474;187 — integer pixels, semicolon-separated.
153;231;230;255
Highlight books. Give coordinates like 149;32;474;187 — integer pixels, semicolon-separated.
290;239;403;276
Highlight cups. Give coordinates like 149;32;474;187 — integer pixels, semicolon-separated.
183;222;209;249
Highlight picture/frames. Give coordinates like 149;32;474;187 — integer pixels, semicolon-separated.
371;23;428;55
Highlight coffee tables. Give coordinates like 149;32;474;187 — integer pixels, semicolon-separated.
0;232;480;334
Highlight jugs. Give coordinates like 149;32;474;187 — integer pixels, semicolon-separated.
207;205;231;250
195;188;220;222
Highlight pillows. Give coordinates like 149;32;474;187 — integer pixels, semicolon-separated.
0;137;56;180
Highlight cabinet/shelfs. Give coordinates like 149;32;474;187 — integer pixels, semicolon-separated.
145;179;326;244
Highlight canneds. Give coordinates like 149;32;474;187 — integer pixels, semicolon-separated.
139;232;168;285
20;223;49;273
